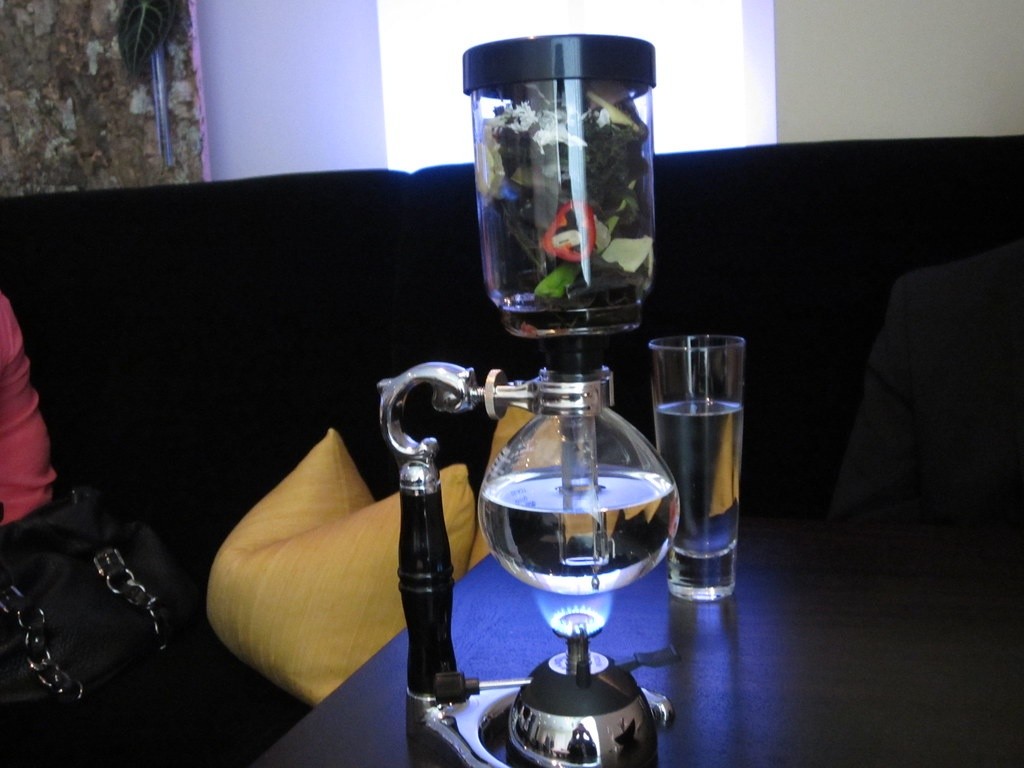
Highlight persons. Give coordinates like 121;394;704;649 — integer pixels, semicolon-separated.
0;289;59;527
827;239;1024;528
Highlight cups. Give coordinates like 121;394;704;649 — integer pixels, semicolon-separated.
648;335;744;601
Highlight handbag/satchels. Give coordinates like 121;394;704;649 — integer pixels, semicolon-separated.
0;486;203;704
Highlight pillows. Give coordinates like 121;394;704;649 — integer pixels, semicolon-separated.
206;404;621;709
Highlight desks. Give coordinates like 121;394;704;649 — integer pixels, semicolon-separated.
249;552;1024;768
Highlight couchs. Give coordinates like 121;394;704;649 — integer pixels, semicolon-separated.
0;135;1024;768
